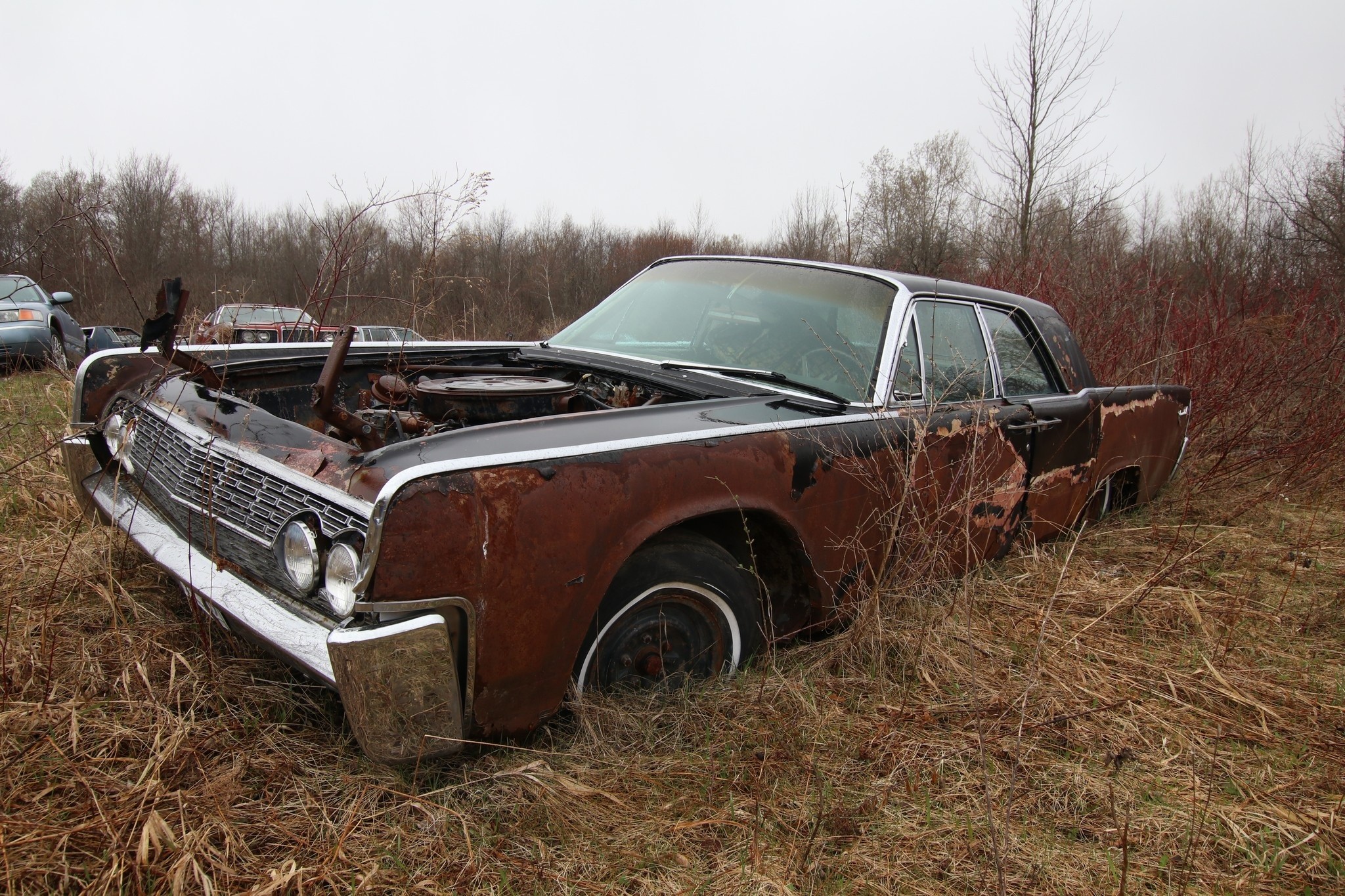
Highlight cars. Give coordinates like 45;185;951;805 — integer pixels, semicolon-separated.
186;303;360;346
0;273;87;371
80;326;190;358
59;255;1193;762
353;326;427;343
